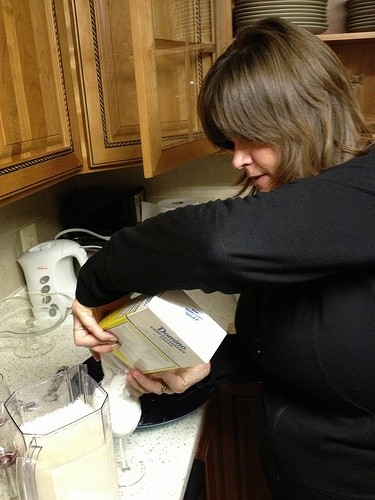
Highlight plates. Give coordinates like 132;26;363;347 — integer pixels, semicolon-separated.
68;356;215;428
346;1;375;32
168;0;211;41
232;0;329;35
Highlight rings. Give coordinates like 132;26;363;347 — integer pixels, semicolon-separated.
72;327;84;331
157;383;167;396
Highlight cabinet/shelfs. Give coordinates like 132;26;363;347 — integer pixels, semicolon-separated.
185;384;269;500
0;0;375;208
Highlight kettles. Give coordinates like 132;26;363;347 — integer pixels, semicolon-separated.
17;240;89;321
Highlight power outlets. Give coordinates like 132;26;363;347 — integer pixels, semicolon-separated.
19;223;39;252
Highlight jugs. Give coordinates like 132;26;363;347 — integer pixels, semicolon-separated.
4;363;120;500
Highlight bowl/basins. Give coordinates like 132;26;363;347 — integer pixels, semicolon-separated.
0;292;73;356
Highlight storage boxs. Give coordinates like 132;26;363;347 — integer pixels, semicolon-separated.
98;291;227;374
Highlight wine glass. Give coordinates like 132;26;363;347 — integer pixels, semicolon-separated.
0;373;24;500
93;379;145;487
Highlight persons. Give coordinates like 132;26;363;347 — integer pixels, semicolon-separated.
71;14;375;500
